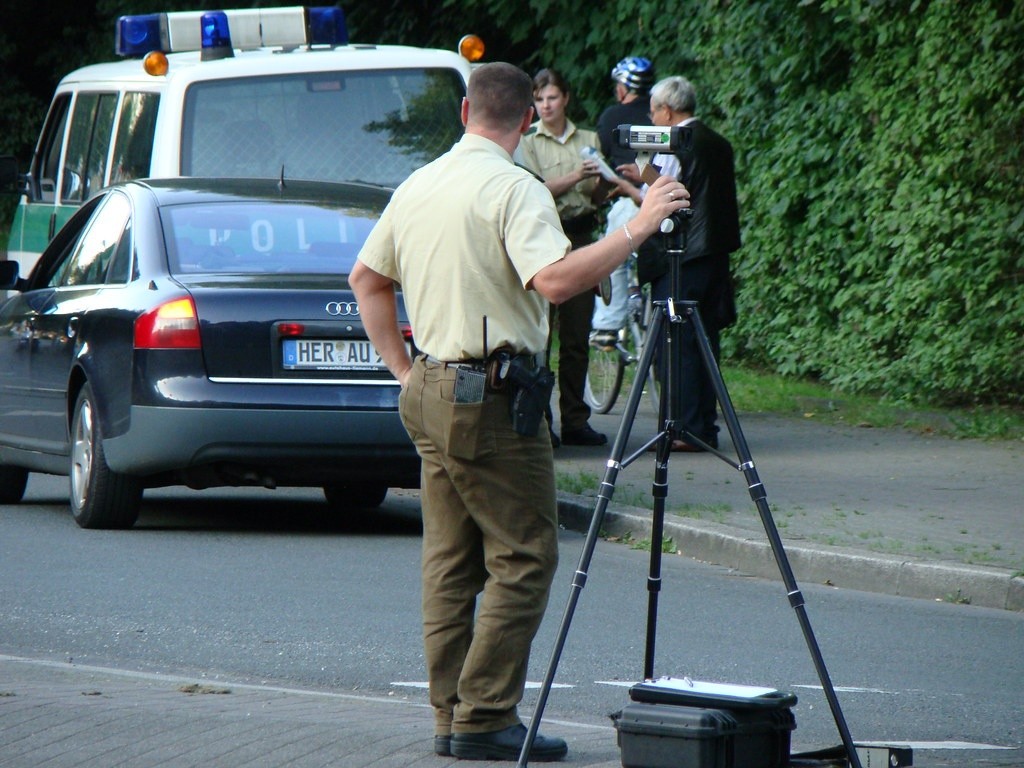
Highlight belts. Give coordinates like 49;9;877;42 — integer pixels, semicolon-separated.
418;352;488;370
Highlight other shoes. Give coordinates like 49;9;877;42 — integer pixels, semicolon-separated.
670;437;720;453
559;420;608;446
641;442;659;452
549;430;561;447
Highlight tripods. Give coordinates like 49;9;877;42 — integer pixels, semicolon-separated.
517;206;862;768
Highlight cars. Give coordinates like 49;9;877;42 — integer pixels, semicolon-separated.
0;175;421;530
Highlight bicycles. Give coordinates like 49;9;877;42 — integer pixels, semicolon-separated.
584;197;636;415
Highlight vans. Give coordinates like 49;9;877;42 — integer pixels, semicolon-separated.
0;5;486;315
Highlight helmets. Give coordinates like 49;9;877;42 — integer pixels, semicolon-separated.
611;55;660;90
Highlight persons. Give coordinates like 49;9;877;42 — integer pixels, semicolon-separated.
607;75;741;451
513;55;655;449
346;62;691;762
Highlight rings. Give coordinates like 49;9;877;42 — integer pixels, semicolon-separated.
669;192;676;199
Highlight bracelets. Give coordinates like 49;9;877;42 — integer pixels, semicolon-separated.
623;224;634;252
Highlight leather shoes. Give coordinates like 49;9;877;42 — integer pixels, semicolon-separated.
450;717;569;761
434;733;452;756
